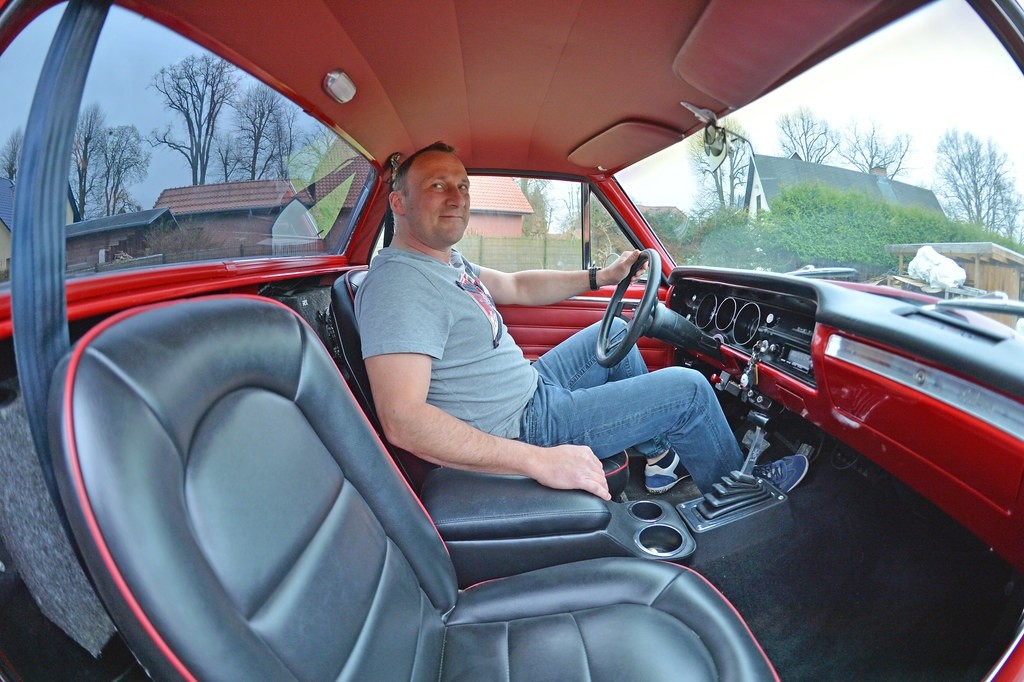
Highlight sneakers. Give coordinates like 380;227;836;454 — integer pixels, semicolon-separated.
644;452;691;493
752;454;809;494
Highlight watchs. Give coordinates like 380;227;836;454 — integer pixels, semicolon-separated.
589;265;601;291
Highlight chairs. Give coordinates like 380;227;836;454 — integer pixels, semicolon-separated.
46;293;783;682
326;268;630;496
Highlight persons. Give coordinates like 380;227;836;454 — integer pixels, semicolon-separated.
354;141;809;501
